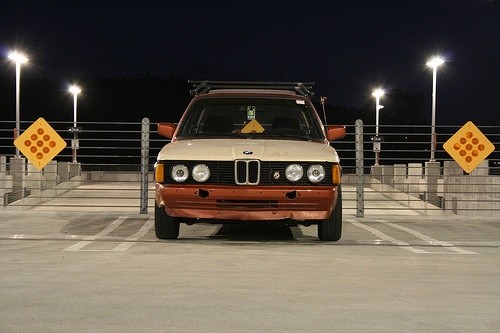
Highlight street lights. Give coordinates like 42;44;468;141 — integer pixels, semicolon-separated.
426;56;444;162
8;53;29;159
371;89;385;166
69;88;81;163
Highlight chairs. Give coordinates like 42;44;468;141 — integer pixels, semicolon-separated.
270;115;304;130
203;115;235;134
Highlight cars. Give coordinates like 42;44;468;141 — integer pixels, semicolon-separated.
155;89;342;242
